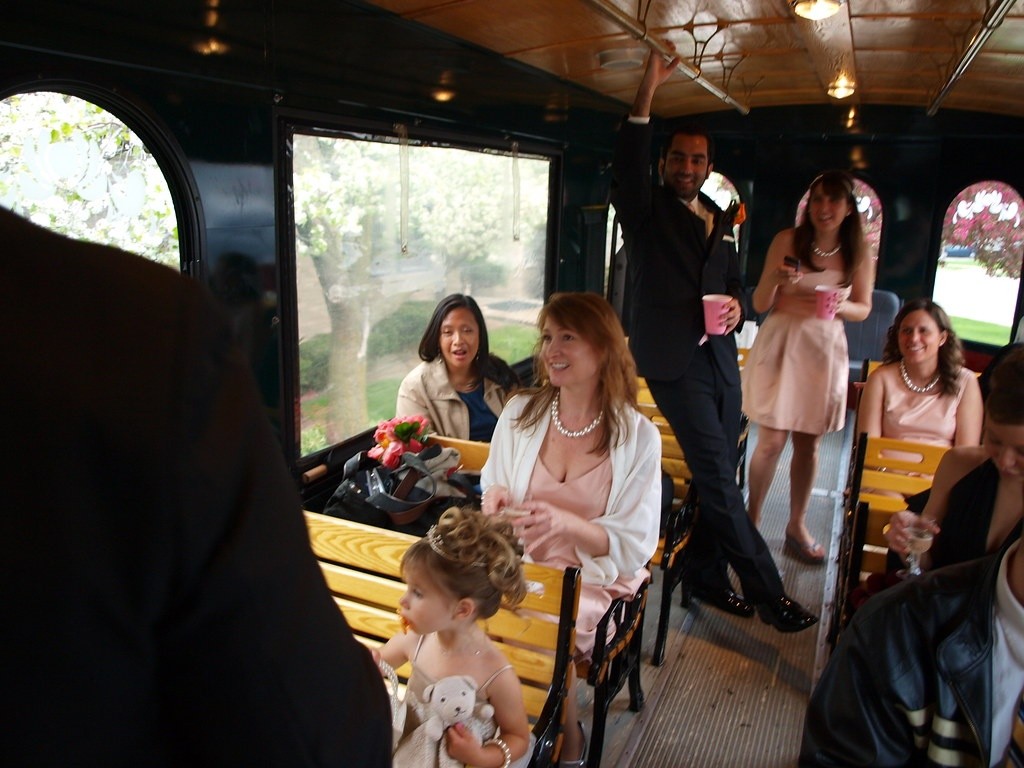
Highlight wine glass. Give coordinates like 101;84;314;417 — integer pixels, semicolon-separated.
897;513;936;580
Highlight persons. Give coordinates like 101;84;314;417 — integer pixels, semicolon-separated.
741;166;872;561
395;292;523;497
368;509;529;768
884;343;1024;574
611;41;821;632
860;300;983;591
0;208;392;768
799;534;1024;768
480;293;662;768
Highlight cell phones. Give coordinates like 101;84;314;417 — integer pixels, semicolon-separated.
784;256;800;273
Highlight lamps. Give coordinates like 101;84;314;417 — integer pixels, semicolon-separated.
791;0;842;20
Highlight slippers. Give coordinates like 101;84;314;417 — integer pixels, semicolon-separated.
785;532;826;563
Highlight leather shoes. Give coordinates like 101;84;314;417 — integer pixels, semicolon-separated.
755;595;820;633
559;720;588;768
689;586;753;617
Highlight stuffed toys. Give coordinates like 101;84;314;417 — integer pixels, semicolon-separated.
422;676;495;768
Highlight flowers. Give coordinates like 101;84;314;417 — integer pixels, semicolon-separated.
365;417;443;463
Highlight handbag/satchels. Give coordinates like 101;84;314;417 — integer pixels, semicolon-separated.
324;445;481;538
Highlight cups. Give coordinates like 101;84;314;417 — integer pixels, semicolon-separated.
702;295;732;334
815;286;839;320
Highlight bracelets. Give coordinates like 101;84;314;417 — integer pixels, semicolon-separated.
480;484;496;505
484;740;511;768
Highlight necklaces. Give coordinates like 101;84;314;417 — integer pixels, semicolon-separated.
551;391;603;438
900;357;941;392
432;631;485;656
810;242;841;258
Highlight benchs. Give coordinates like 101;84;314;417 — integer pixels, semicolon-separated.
827;292;983;642
306;359;750;768
